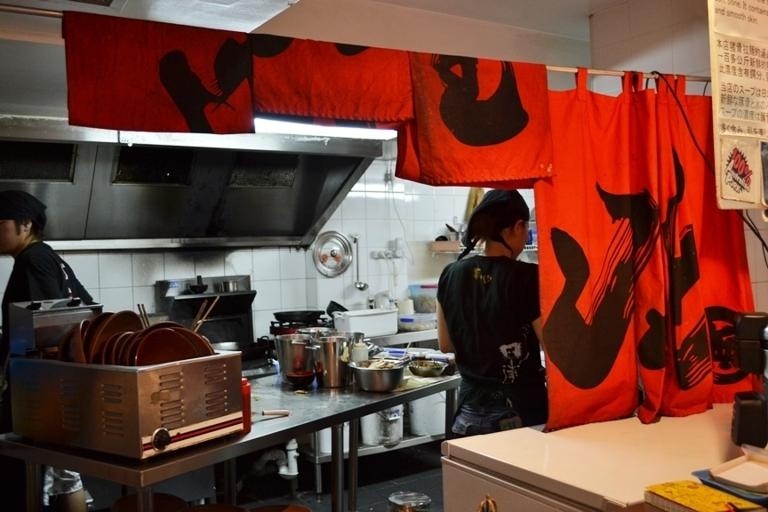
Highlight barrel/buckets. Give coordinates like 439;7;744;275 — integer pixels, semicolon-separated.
407;391;446;437
276;325;364;388
360;404;405;446
312;422;350;454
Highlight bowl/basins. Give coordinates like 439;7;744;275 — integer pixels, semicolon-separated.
191;284;208;293
410;360;449;376
348;359;404;392
286;371;315;388
222;280;237;293
67;311;213;371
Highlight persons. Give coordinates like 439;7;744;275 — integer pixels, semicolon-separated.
436;189;548;439
0;190;93;512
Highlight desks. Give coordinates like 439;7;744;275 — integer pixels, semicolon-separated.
301;328;462;505
0;373;463;512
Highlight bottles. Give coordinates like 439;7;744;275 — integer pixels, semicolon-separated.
240;378;251;436
351;333;369;363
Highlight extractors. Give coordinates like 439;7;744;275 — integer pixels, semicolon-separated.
0;42;385;251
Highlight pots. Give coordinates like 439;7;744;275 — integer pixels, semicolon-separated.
274;301;348;322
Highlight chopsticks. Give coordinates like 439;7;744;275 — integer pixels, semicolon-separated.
190;295;221;333
137;304;151;328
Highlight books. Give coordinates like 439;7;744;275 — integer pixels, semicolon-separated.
643;478;763;512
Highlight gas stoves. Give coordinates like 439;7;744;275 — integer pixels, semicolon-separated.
268;319;333;336
